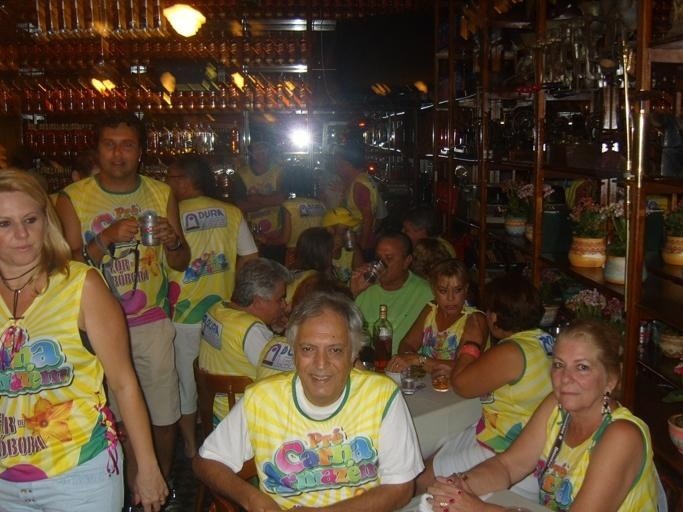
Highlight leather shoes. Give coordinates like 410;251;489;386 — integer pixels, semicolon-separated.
162;488;177;511
122;497;143;512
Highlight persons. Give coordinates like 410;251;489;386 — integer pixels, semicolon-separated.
0;134;555;496
191;292;426;511
0;171;168;512
426;318;668;512
54;112;190;510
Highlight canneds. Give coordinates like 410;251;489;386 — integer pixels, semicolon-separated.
140;213;160;246
345;230;356;251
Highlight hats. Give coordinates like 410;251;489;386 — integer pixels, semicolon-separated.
252;130;274;149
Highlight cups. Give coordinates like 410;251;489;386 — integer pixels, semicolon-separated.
431;377;450;392
399;367;418;396
364;255;388;284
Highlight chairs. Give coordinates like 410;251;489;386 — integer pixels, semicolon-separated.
190;354;258;512
206;458;260;512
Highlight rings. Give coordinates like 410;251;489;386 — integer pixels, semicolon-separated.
439;498;450;509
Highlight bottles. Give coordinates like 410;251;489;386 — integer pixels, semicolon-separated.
23;81;312;115
372;302;394;370
145;121;240;155
15;32;309;66
25;121;99;152
